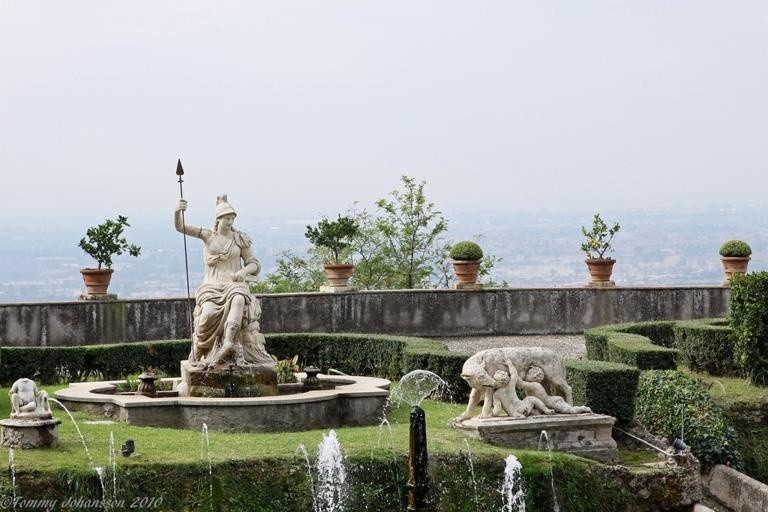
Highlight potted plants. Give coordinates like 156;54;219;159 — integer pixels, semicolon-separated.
579;213;620;287
78;215;142;301
303;213;360;292
719;240;752;282
449;241;484;289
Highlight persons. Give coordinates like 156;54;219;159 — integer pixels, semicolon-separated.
174;194;275;369
490;359;594;418
8;378;37;415
